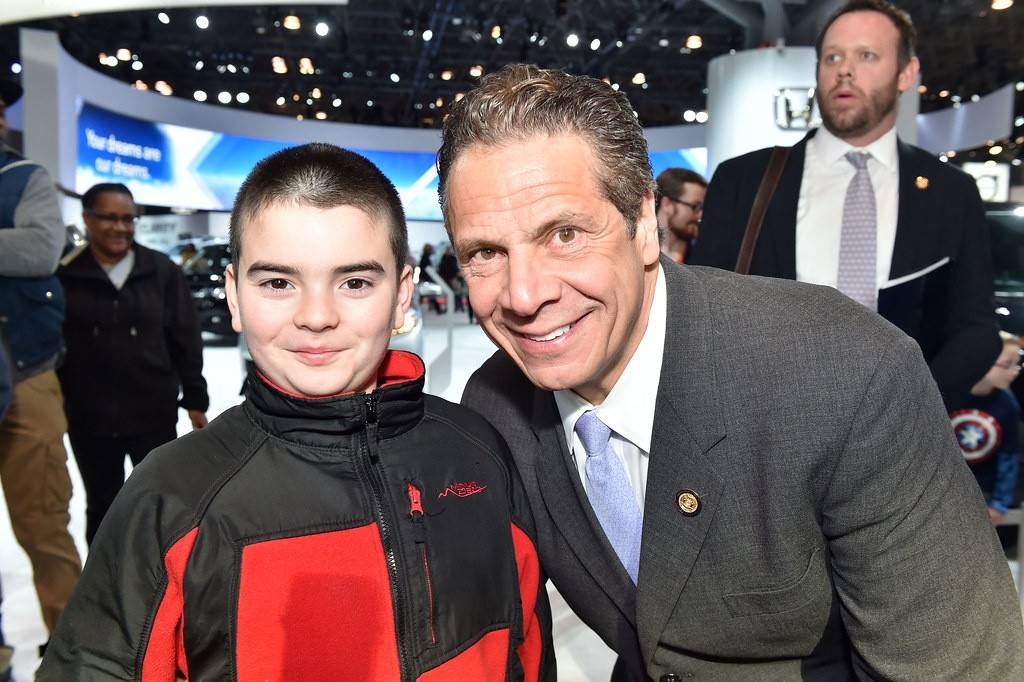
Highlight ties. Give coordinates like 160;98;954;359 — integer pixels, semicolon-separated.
837;152;878;313
573;413;644;587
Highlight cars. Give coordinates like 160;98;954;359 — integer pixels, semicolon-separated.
182;242;233;335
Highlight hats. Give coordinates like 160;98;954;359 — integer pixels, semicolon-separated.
423;244;436;254
179;243;197;255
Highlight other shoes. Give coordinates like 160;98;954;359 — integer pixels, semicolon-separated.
0;644;16;682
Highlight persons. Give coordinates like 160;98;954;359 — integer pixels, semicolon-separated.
439;64;1024;682
654;168;708;263
33;142;558;682
945;331;1024;526
0;102;82;682
419;243;480;324
55;182;210;552
698;0;1004;409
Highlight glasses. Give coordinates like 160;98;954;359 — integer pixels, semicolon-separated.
655;195;704;213
88;210;134;223
